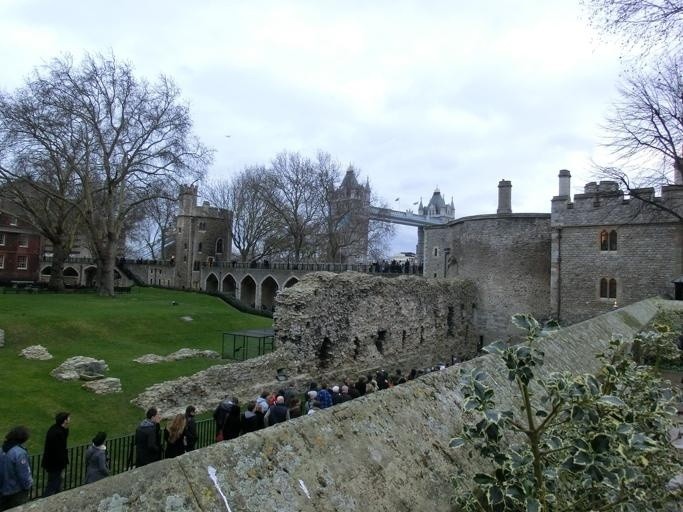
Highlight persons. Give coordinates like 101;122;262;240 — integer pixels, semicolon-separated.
162;412;193;459
0;425;33;511
180;405;198;452
133;408;163;468
213;359;459;444
84;430;112;486
371;258;423;275
37;410;70;498
